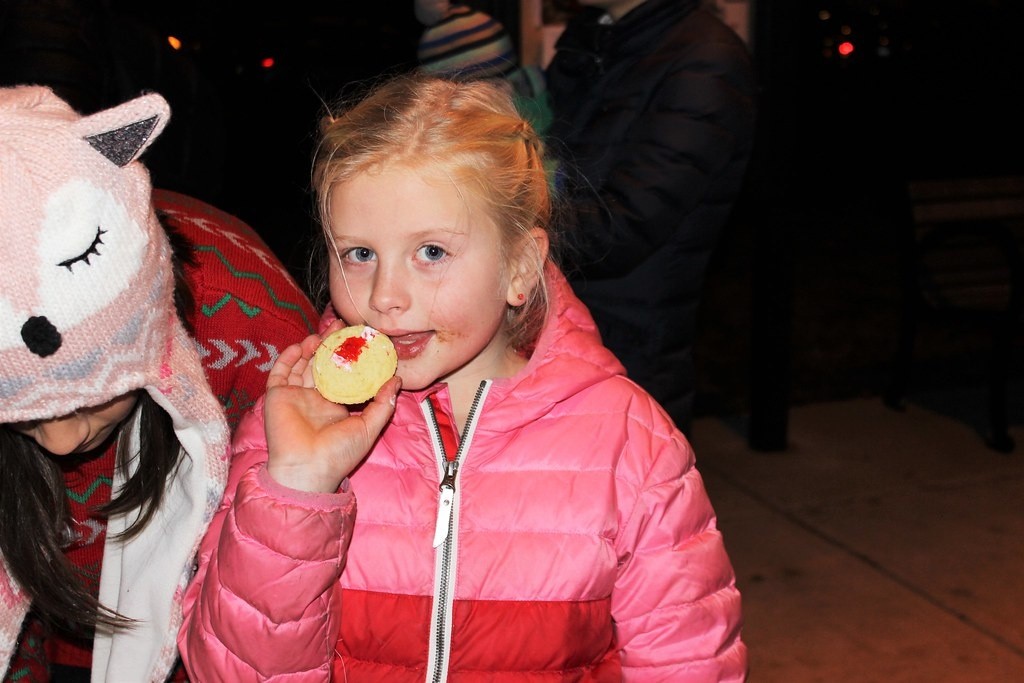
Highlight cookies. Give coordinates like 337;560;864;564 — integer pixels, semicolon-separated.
311;325;398;404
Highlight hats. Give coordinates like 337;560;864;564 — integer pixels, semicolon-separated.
415;0;517;83
0;84;231;683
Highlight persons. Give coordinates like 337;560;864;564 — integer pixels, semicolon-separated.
179;77;750;683
544;0;748;441
0;82;327;683
